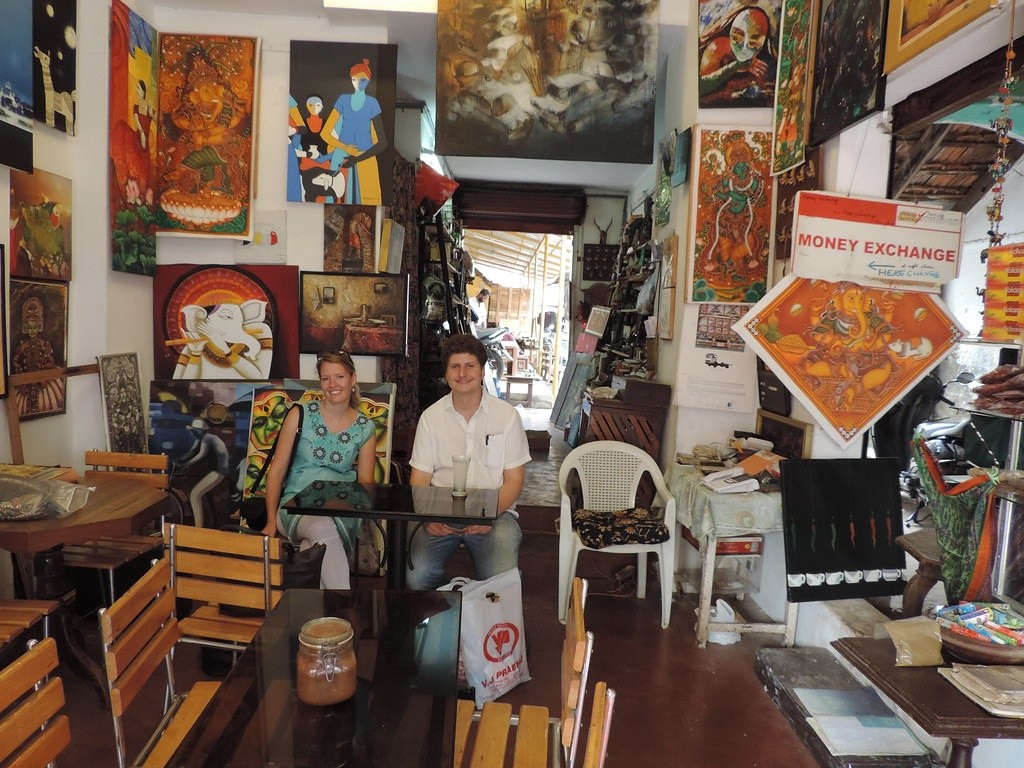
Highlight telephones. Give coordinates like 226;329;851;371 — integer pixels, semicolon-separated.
699;466;760;494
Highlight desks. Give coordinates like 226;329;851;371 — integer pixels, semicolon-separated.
159;586;462;768
829;636;1024;768
0;472;168;712
664;461;797;649
280;478;499;595
502;373;535;408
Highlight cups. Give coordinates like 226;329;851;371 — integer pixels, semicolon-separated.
452;455;472;498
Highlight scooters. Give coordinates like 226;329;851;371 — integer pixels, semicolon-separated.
870;372;975;525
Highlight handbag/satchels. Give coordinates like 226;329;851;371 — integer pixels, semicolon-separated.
457;566;531;709
413;576;474;681
695;599;741;645
239;496;267;530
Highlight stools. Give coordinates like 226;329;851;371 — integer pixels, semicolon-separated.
895;527;948;620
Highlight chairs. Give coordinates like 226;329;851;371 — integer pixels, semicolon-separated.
558;440;677;630
159;521;285;717
61;447;176;606
454;578;616;768
0;597;71;768
97;556;225;767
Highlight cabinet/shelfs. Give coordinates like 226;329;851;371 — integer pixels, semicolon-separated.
578;390;665;460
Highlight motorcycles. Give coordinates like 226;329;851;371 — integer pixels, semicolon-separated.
478;328;514;381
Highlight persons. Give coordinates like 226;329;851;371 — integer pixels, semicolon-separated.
261;350;376;588
406;340;533;590
469;289;490;333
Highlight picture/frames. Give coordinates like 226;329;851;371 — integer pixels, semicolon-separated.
808;0;889;149
298;270;412;359
756;408;814;460
883;0;999;74
96;353;151;474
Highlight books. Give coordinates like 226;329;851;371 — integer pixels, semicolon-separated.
738;450;787;478
936;665;1024;719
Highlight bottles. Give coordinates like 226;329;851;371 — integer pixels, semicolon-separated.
295;617;356;706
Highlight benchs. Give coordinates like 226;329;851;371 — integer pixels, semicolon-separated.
751;645;934;768
